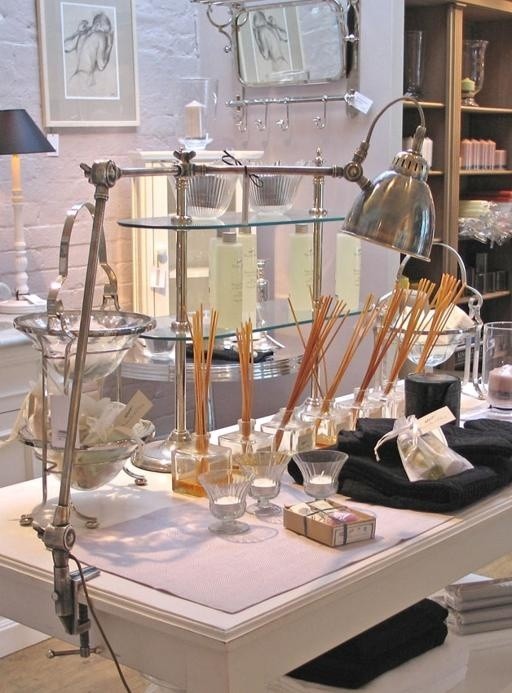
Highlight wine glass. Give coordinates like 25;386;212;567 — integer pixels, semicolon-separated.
461;40;488;107
197;449;349;535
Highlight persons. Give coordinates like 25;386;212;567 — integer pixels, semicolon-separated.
249;10;290;73
64;11;115;88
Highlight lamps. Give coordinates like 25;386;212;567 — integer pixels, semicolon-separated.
401;0;512;379
0;106;59;314
20;94;436;659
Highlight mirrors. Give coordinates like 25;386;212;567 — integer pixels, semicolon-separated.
230;0;347;88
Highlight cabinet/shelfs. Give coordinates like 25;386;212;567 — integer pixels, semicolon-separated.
118;148;370;474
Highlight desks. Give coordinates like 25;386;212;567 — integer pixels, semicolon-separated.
2;374;512;692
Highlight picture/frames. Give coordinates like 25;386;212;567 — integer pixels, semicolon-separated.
34;0;144;131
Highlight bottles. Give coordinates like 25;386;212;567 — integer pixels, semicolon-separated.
289;224;361;312
207;224;258;331
170;380;404;498
400;427;461;481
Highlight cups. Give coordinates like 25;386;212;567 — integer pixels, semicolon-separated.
474;250;506;293
404;30;426;99
481;321;511;409
